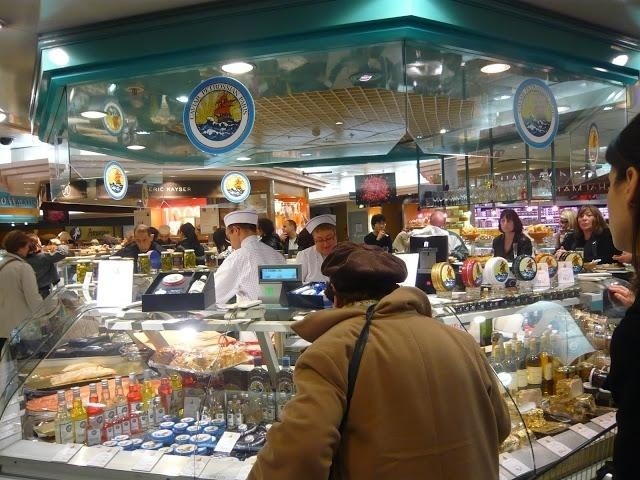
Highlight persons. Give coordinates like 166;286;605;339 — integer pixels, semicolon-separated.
91;222;205;274
0;230;82;362
296;213;336;286
247;241;512;480
553;204;636;308
202;210;299;309
587;114;637;479
364;214;392;256
392;211;470;272
493;209;532;288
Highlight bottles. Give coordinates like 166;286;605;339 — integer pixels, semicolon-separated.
204;388;290;428
188;275;208;293
55;367;183;446
245;356;272;394
275;356;297;421
489;336;555;402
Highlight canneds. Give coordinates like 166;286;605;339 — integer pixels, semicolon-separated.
184;249;197;269
76;254;121;283
171;251;184;270
137;254;151;274
160;251;172;271
202;251;218;267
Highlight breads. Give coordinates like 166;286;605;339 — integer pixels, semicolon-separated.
154;344;245;370
81;243;110;255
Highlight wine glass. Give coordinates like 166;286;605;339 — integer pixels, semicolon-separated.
431;183;493;206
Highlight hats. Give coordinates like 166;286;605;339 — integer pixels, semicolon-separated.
304;214;336;234
223;211;259;229
159;225;170;235
321;241;408;283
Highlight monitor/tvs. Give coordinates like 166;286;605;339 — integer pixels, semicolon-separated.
393;253;419;288
409;235;448;270
